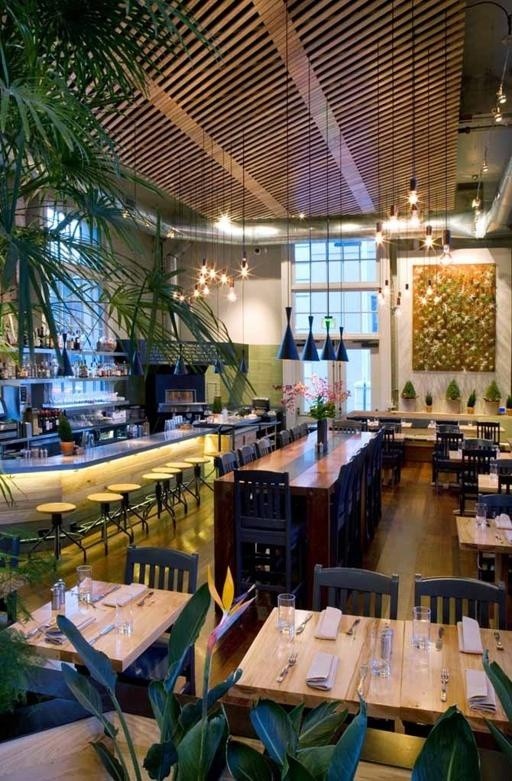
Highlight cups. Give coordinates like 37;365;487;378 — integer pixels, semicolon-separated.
77;565;91;599
475;502;484;528
413;608;430;649
278;594;295;633
116;593;131;632
126;425;137;448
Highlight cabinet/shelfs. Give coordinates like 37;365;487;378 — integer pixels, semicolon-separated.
0;346;149;460
0;413;281;544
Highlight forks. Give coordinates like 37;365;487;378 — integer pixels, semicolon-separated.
440;667;449;701
276;649;298;682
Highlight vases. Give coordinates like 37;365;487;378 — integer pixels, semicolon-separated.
317;419;327;453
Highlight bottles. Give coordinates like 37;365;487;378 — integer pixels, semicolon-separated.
49;584;60;610
56;578;65;604
39;360;50;377
72;360;88;378
35;327;80;350
39;409;60;433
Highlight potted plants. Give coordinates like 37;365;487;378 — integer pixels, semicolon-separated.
446;378;461;404
466;390;476;414
482;380;502;410
401;381;416;405
425;392;433;412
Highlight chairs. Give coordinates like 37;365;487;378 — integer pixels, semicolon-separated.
237;443;258;466
0;532;21;626
279;429;290;446
459;449;498;516
378;418;402;434
476;492;512;581
435;420;459;437
463;438;493;450
75;545;200;697
430;432;464;486
299;422;309;438
232;467;306;607
255;437;273;458
346;415;369;432
402;573;506;739
476;421;501;445
331;437;383;567
289;425;301;442
379;428;402;485
215;450;242;478
311;563;400;732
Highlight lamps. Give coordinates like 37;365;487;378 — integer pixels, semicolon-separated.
320;320;336;361
336;326;349;362
174;358;188;376
384;279;390;295
497;94;507;104
201;258;207;273
493;113;503;123
408;178;419;204
240;360;248;373
277;307;300;361
227;277;237;302
213;359;226;374
410;205;420;228
194;284;199;296
442;229;450;254
179;290;184;301
388;204;399;231
128;350;145;376
426;279;433;295
210;263;216;278
404;283;410;298
199;273;205;284
377;287;383;301
240;259;248;277
394;297;402;318
221;269;227;283
374;222;384;243
300;315;320;362
425;225;433;246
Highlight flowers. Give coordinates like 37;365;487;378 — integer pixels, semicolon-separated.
277;374;352;420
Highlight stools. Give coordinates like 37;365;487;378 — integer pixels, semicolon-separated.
28;502;86;571
142;473;177;531
182;457;215;500
79;492;132;555
101;483;148;543
166;461;200;508
152;467;187;517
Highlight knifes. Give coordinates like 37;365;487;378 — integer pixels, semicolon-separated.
435;627;446;651
296;612;312;633
